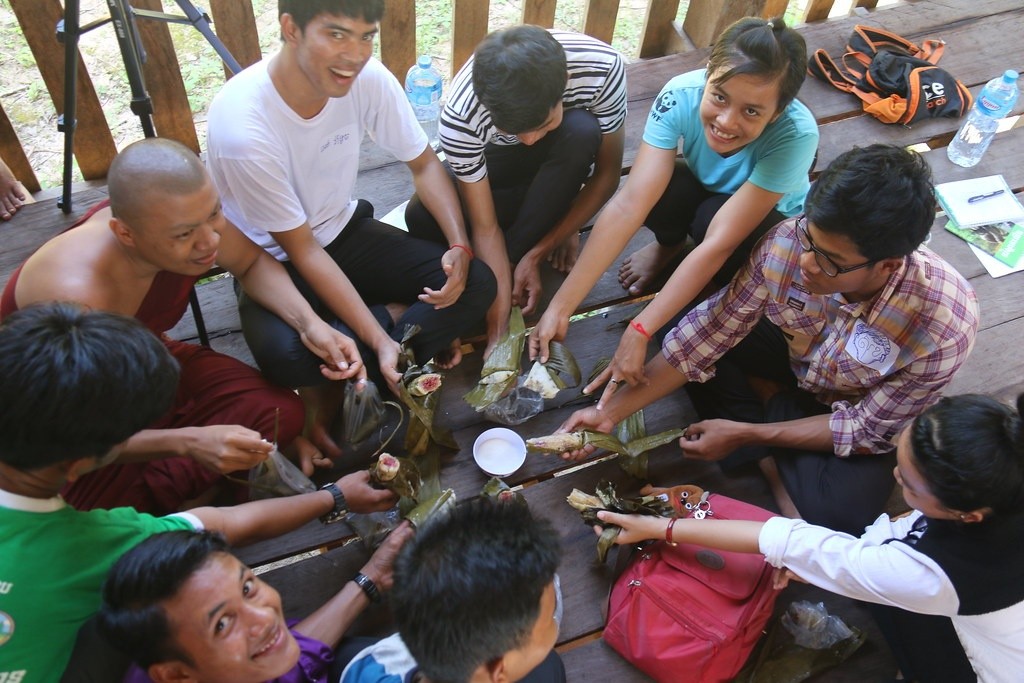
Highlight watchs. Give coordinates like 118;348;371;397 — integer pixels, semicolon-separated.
317;482;348;524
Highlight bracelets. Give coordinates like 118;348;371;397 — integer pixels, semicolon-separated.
666;517;678;546
349;571;381;604
630;320;652;341
451;245;472;257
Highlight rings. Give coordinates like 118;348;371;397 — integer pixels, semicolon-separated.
610;379;618;384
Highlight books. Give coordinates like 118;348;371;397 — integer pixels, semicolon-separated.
934;174;1024;278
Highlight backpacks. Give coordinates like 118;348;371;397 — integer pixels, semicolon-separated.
806;23;973;127
601;489;791;683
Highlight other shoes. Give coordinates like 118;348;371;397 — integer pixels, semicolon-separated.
433;336;463;368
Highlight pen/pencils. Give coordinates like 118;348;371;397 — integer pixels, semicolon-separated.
968;190;1004;203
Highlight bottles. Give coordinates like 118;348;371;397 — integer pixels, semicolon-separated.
947;69;1020;167
405;56;443;152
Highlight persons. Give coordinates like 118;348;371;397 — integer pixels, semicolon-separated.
528;17;822;410
0;299;403;683
594;383;1023;683
102;521;419;682
405;24;627;404
0;137;366;513
0;158;25;220
208;0;497;452
340;492;569;683
540;142;978;539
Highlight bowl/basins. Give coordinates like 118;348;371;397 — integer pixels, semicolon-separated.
473;428;528;479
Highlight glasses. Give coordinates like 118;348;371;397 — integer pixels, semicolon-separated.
794;213;884;277
